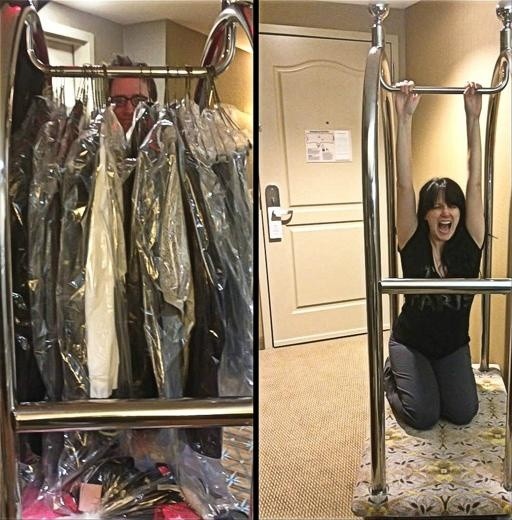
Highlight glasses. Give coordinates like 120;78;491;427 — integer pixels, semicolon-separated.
106;93;155;108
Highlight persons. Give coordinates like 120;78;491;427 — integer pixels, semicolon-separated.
105;53;158;132
383;78;487;430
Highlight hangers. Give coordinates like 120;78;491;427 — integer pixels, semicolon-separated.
12;65;252;166
